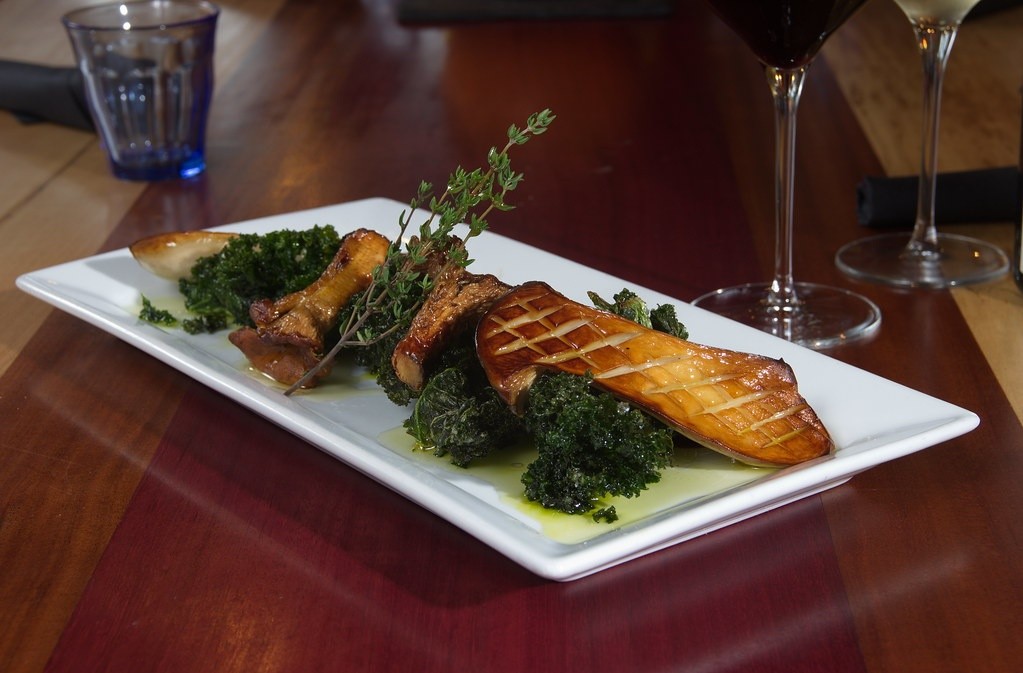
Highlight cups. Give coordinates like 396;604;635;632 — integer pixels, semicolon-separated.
60;0;221;181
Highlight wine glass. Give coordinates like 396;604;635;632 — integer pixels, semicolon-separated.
688;0;882;350
835;0;1011;290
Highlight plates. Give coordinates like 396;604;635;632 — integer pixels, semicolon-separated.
14;197;982;585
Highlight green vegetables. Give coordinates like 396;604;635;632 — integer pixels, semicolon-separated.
136;107;691;527
